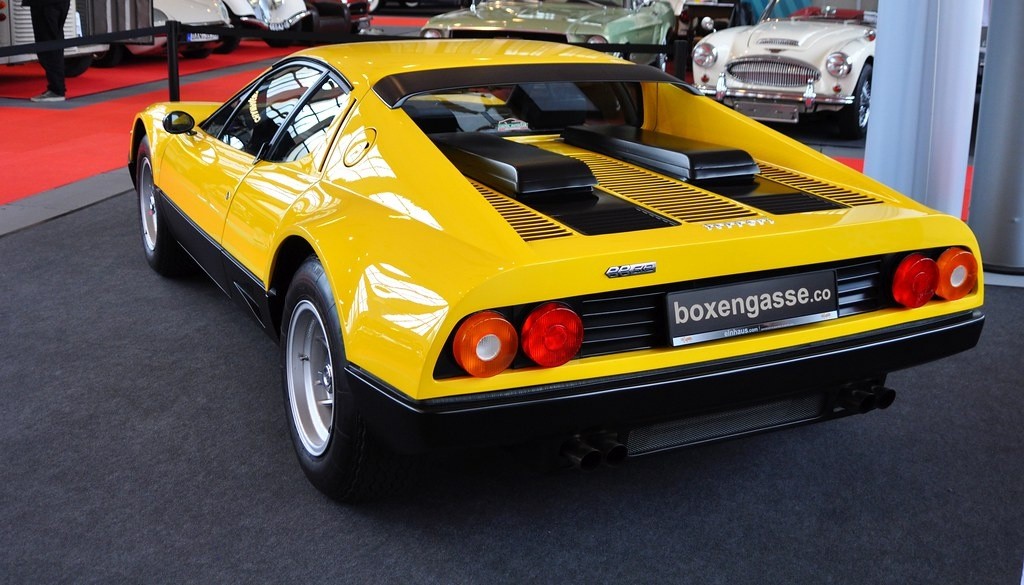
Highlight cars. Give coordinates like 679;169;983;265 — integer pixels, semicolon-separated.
693;0;879;140
237;0;372;49
420;0;680;72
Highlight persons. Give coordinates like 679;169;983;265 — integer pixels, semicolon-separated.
20;0;70;103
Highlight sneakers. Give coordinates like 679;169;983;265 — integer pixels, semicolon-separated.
30;90;66;103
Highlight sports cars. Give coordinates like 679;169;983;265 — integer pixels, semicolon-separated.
128;37;985;507
